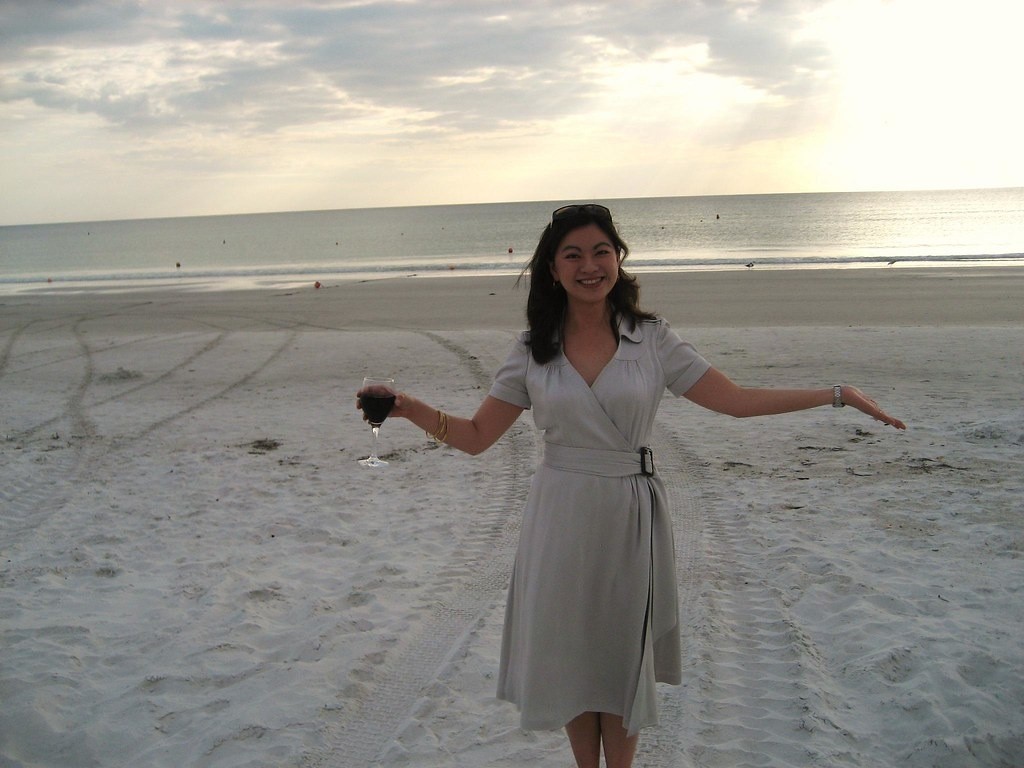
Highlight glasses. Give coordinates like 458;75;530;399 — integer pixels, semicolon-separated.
550;204;613;235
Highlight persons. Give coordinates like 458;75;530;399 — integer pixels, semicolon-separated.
356;204;906;768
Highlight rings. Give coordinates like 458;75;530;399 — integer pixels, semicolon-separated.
873;409;882;422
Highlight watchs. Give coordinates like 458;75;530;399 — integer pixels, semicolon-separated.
832;384;846;408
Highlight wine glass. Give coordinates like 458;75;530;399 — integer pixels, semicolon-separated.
357;376;397;468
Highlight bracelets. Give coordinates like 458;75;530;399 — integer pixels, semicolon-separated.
426;410;449;443
